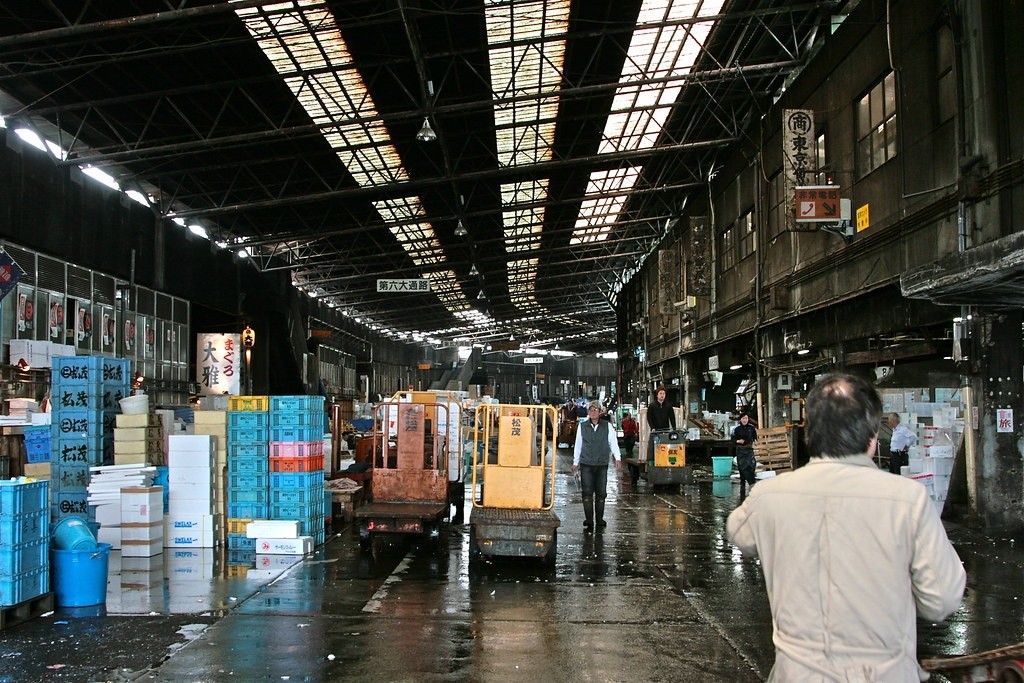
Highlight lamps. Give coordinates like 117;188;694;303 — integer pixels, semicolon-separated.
454;219;468;235
477;290;486;299
416;114;436;140
469;263;479;275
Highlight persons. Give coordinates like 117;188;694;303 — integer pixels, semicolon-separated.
725;371;966;683
533;403;553;455
647;387;677;432
320;378;332;404
622;413;639;458
577;402;588;417
342;431;365;460
888;413;918;475
730;413;757;501
557;400;577;420
573;400;621;525
600;407;613;425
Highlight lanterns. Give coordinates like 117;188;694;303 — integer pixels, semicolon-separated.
243;327;255;348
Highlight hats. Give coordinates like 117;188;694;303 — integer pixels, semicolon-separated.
589;401;601;409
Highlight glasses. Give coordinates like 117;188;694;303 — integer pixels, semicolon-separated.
589;408;600;412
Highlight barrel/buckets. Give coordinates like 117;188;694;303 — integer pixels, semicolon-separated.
711;457;733;476
50;543;113;607
713;482;732;497
119;395;149;414
52;516;97;550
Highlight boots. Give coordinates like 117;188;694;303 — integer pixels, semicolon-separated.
595;498;606;526
583;496;594;526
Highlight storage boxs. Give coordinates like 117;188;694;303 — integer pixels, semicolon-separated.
654;443;686;468
0;355;545;625
